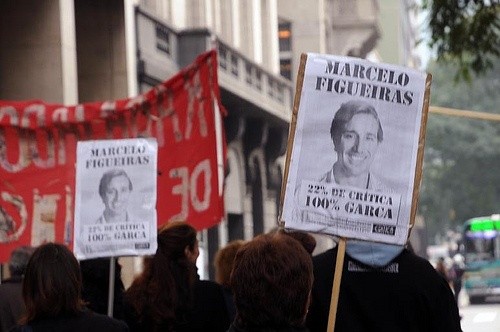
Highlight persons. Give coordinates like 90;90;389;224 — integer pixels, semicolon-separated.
0;222;468;332
93;168;138;222
294;99;406;226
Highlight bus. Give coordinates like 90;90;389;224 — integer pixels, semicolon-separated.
464;215;500;303
